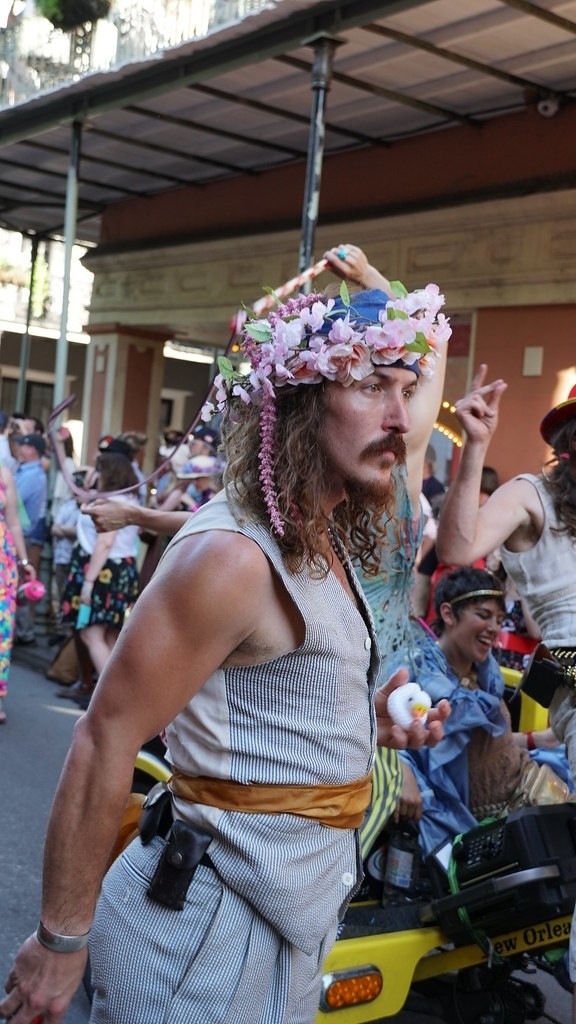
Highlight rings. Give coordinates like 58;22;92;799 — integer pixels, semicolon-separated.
338;252;348;259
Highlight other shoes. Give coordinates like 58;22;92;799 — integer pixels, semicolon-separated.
0;712;6;720
13;636;37;647
48;634;65;646
56;680;94;697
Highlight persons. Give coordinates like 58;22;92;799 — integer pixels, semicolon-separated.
0;285;451;1024
437;361;576;798
0;413;576;854
0;459;36;723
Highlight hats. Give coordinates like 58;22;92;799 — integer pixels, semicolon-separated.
99;436;132;461
177;455;224;479
540;383;576;445
193;426;219;447
12;434;45;452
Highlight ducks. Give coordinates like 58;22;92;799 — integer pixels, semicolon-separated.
385;682;433;732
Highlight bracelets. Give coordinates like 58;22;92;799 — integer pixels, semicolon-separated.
36;922;92;952
527;731;536;751
17;558;30;566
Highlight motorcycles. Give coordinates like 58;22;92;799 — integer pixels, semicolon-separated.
114;734;576;1024
